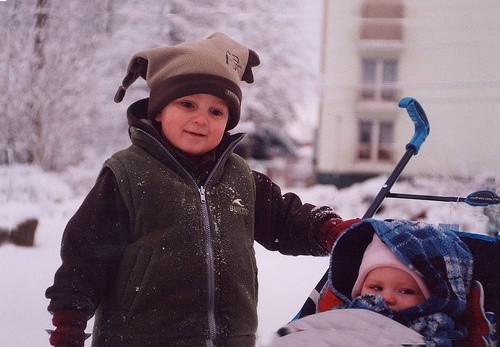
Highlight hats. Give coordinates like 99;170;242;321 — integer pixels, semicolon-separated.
350;232;433;302
114;32;261;130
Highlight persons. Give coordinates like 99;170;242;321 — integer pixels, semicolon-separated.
313;216;478;346
44;32;361;347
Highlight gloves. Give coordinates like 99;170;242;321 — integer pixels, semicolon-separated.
44;314;90;347
318;217;362;249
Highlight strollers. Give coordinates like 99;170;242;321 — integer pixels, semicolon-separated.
277;97;500;347
